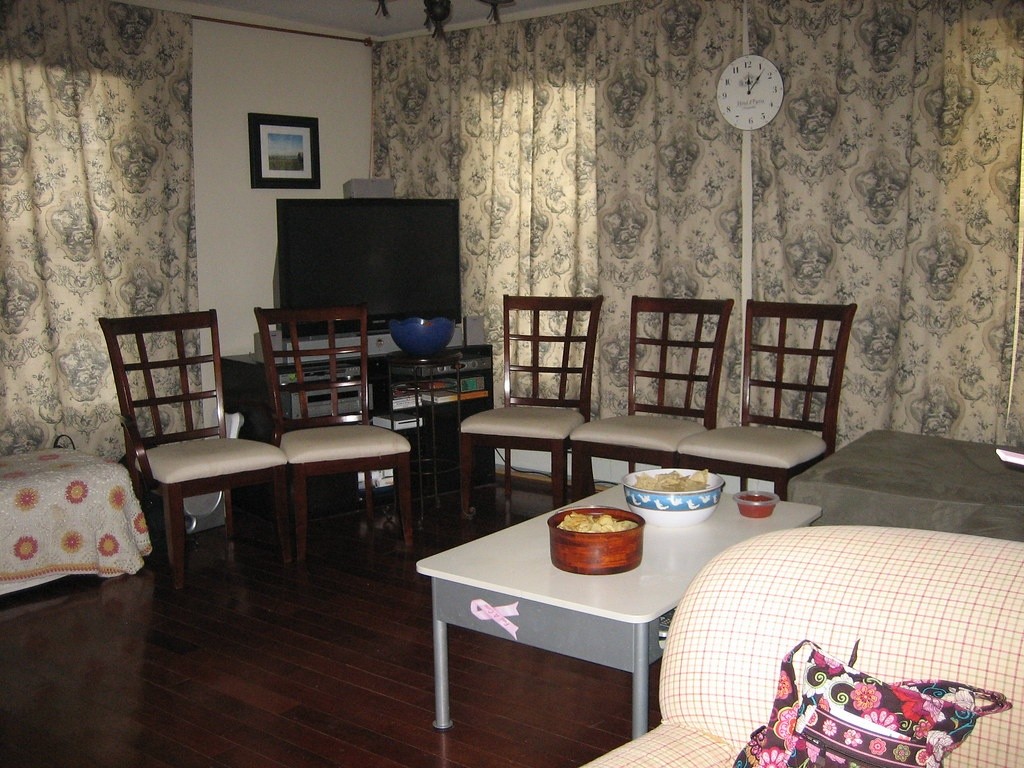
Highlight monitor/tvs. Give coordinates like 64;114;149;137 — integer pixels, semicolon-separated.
276;198;463;364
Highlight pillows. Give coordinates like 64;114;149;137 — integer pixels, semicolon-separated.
726;640;1013;768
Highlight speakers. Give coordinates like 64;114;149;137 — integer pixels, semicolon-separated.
254;331;283;364
463;315;485;346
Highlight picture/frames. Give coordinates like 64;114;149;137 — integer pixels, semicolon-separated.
247;111;322;190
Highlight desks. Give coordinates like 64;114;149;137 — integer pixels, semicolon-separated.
0;446;153;598
383;350;465;522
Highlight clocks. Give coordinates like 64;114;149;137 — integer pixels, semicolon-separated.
715;51;787;133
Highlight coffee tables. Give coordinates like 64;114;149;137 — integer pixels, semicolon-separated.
415;483;823;739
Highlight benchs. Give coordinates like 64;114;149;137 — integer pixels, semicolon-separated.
787;428;1024;543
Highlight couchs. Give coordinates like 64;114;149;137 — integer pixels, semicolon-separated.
574;525;1024;768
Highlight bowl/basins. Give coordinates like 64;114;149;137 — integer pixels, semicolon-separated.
733;491;779;519
548;508;646;575
622;468;725;527
390;318;455;357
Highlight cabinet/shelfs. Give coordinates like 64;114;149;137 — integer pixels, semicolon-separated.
224;346;501;547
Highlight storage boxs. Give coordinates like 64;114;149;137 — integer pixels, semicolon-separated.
342;177;395;199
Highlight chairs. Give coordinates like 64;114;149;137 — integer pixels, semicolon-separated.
569;294;736;503
459;292;604;537
675;298;859;504
100;309;290;585
252;304;417;568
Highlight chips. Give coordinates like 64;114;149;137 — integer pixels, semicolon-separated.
631;467;709;492
557;511;638;532
